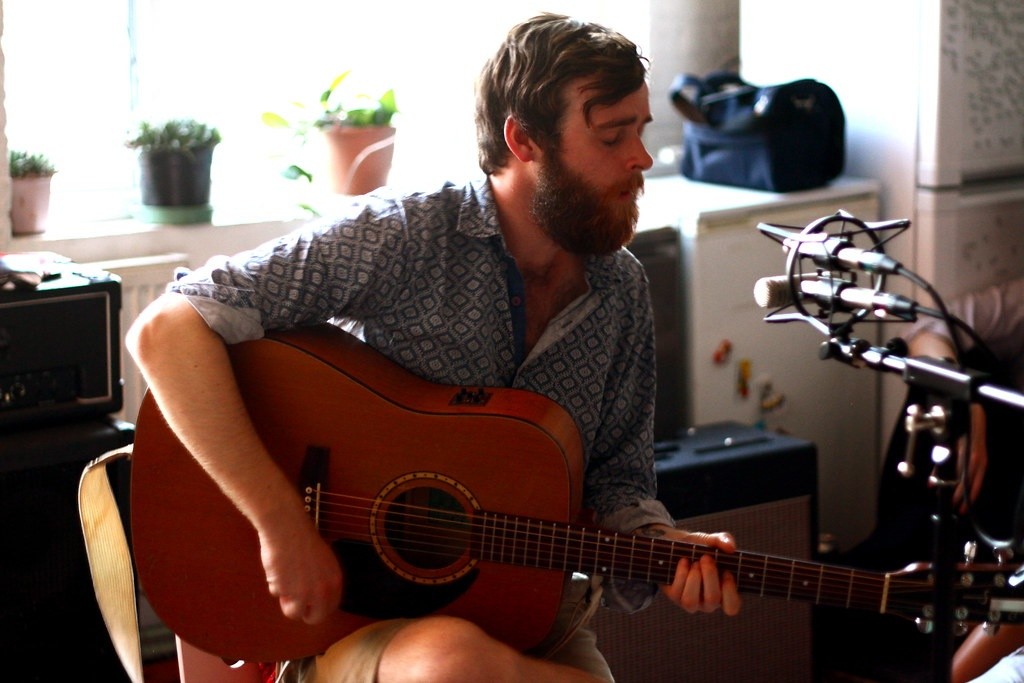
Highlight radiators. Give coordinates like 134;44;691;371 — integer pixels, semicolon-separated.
80;252;189;424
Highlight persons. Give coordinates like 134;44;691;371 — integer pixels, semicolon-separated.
122;13;745;683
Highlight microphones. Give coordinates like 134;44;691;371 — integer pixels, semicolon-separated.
752;211;918;339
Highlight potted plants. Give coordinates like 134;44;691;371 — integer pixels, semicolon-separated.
7;150;59;235
263;65;396;216
124;116;222;224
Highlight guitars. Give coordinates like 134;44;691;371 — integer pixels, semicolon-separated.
128;321;1024;661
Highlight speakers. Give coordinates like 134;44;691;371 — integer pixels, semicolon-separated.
585;418;820;683
0;416;139;683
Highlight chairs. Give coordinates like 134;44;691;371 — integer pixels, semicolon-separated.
176;633;261;683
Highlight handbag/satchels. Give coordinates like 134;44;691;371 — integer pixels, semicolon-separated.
668;71;847;193
